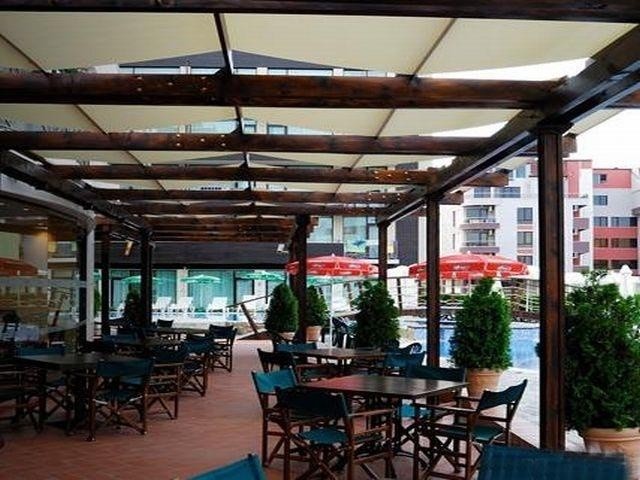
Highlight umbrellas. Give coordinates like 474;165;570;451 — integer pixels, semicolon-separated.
181;274;222;306
409;251;530;296
286;254;377;350
122;275;161;284
238;271;285;281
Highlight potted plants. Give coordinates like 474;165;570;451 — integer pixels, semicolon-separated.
264;283;295;347
533;266;639;480
306;285;330;341
448;278;511;400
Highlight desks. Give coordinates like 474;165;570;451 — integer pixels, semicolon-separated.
291;347;390;376
304;374;468;478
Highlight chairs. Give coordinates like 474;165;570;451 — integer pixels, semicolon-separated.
170;340;210;396
251;365;322;468
276;341;324;381
187;453;269;479
256;347;299;381
423;379;528;478
117;344;181;419
380;351;426;377
208;327;238;371
68;358;150;442
392;365;464;456
480;443;631;480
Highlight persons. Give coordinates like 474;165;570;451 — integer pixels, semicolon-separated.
124;284;138;307
